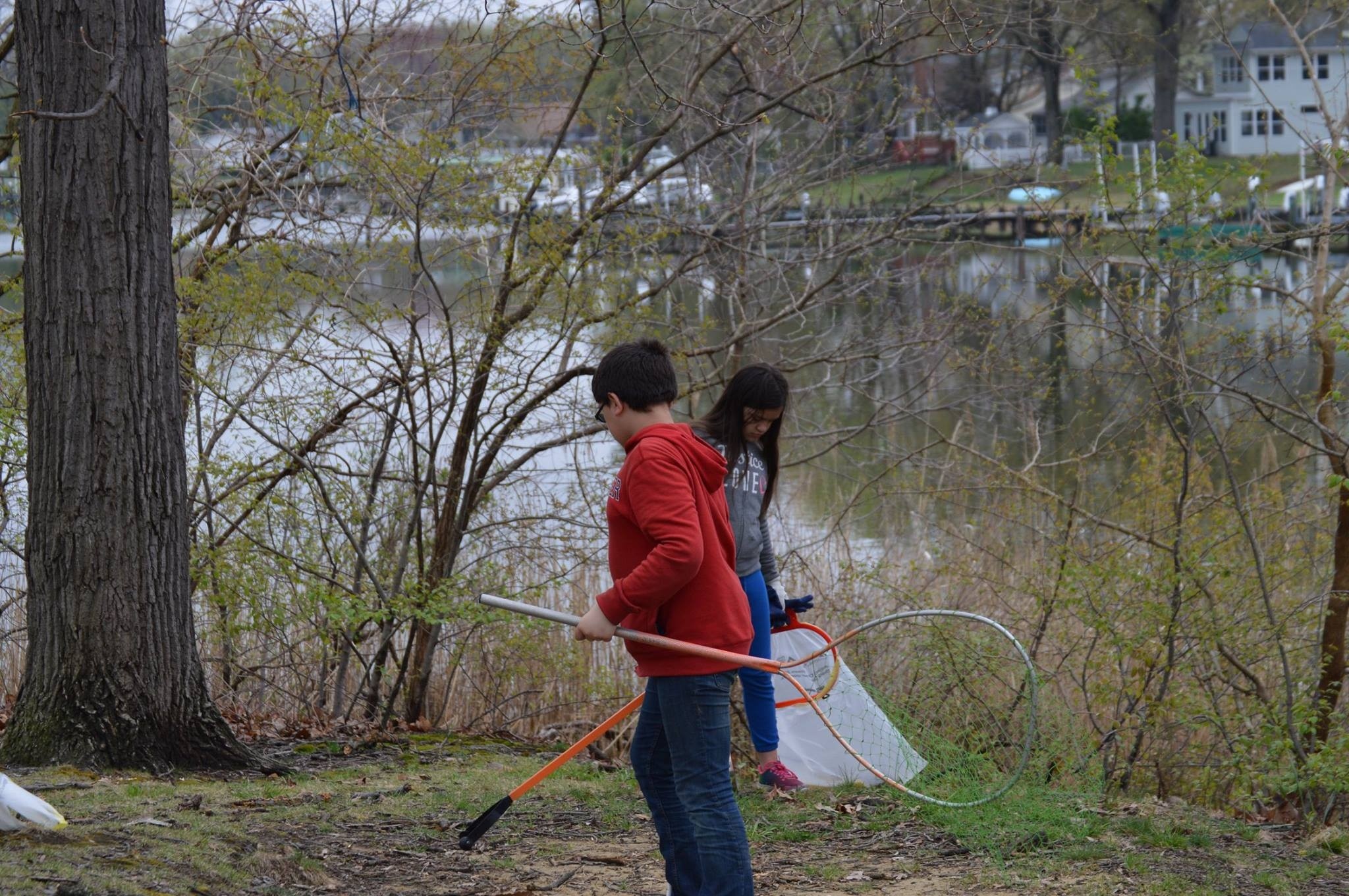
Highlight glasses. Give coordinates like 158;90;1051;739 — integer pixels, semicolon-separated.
594;398;609;423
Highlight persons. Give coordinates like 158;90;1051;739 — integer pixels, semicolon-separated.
574;338;756;896
691;363;807;793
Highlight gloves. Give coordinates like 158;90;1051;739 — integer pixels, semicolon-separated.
764;578;815;628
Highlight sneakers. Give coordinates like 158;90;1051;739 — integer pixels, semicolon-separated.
756;759;806;793
